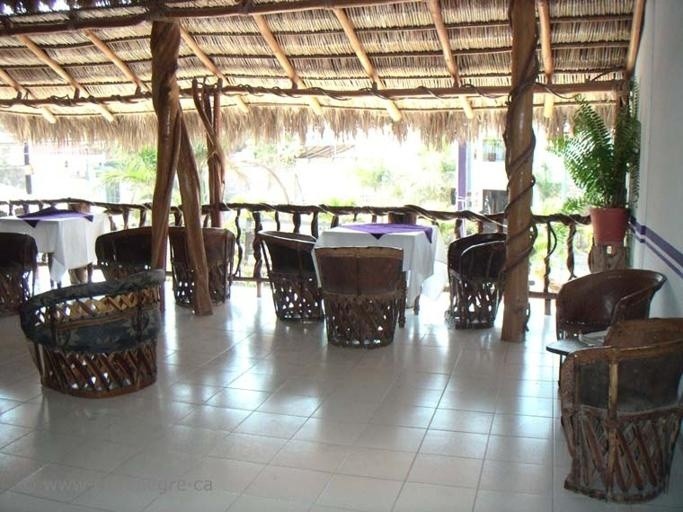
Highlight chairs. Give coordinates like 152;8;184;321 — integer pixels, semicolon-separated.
555;270;667;346
168;226;235;308
315;247;408;348
259;230;324;322
19;270;166;400
558;317;683;503
95;225;154;282
1;232;38;318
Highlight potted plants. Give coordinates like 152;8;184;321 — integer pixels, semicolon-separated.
552;76;641;246
447;233;507;330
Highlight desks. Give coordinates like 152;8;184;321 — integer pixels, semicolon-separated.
311;223;436;326
546;333;608;425
0;207;94;289
